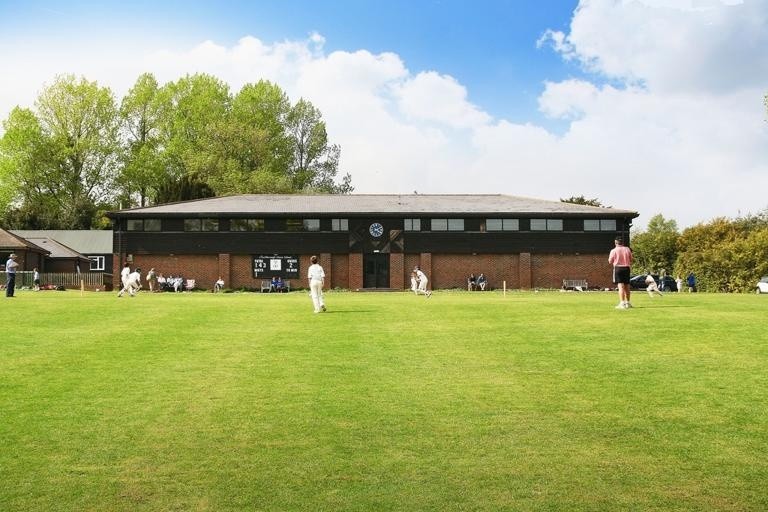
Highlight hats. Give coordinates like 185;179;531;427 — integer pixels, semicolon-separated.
8;254;18;258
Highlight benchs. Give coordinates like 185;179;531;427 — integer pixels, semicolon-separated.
154;277;195;292
259;280;292;293
562;280;588;290
465;278;489;290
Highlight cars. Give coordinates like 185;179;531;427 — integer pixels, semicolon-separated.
753;275;767;295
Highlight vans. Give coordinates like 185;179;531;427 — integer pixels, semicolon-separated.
628;273;676;292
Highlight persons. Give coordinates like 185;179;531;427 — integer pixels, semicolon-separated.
118;262;183;297
6;254;20;297
307;255;326;312
468;273;477;291
277;277;285;293
268;276;278;293
476;273;487;291
32;268;40;291
410;273;420;295
413;265;432;297
608;236;636;307
644;265;695;297
214;276;224;292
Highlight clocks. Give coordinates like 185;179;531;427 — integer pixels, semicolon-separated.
369;222;384;237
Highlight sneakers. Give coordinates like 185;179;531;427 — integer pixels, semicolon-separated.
428;292;432;297
615;303;631;309
321;305;327;311
